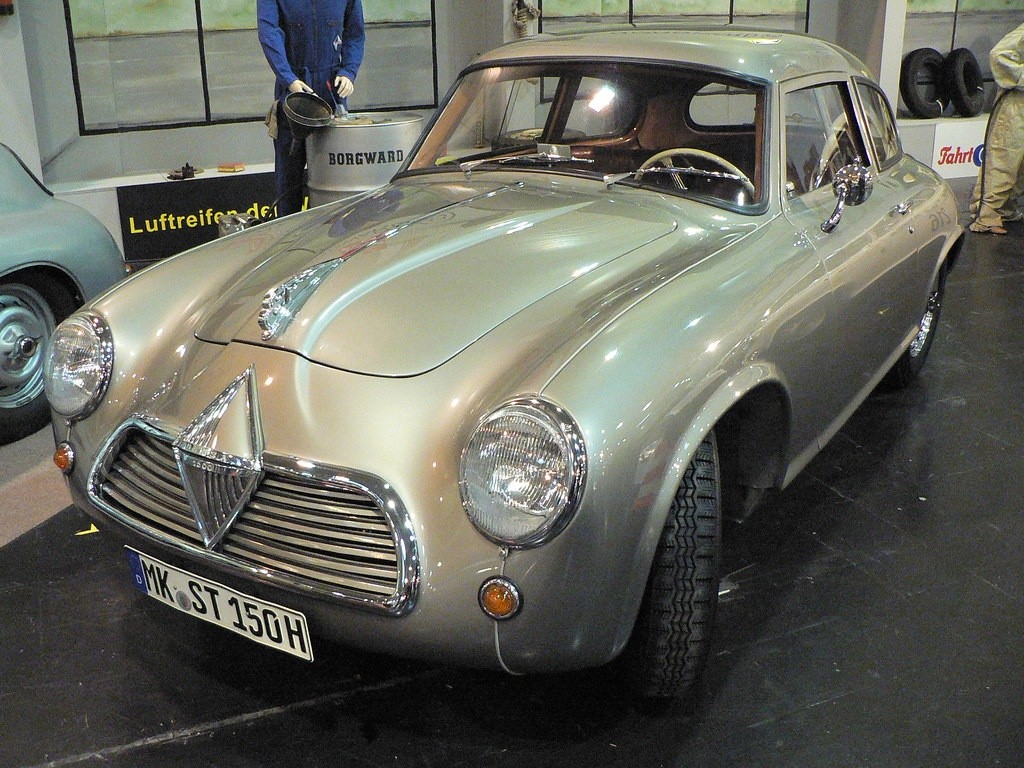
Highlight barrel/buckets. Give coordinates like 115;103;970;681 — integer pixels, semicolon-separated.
305;110;426;208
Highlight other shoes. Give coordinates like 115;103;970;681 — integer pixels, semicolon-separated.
1001;210;1024;222
969;221;1007;233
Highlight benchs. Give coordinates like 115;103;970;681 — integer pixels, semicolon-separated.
551;146;757;207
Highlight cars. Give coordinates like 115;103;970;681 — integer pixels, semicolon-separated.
1;142;125;439
41;27;969;703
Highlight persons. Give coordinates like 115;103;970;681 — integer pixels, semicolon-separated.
257;0;365;219
970;20;1024;236
